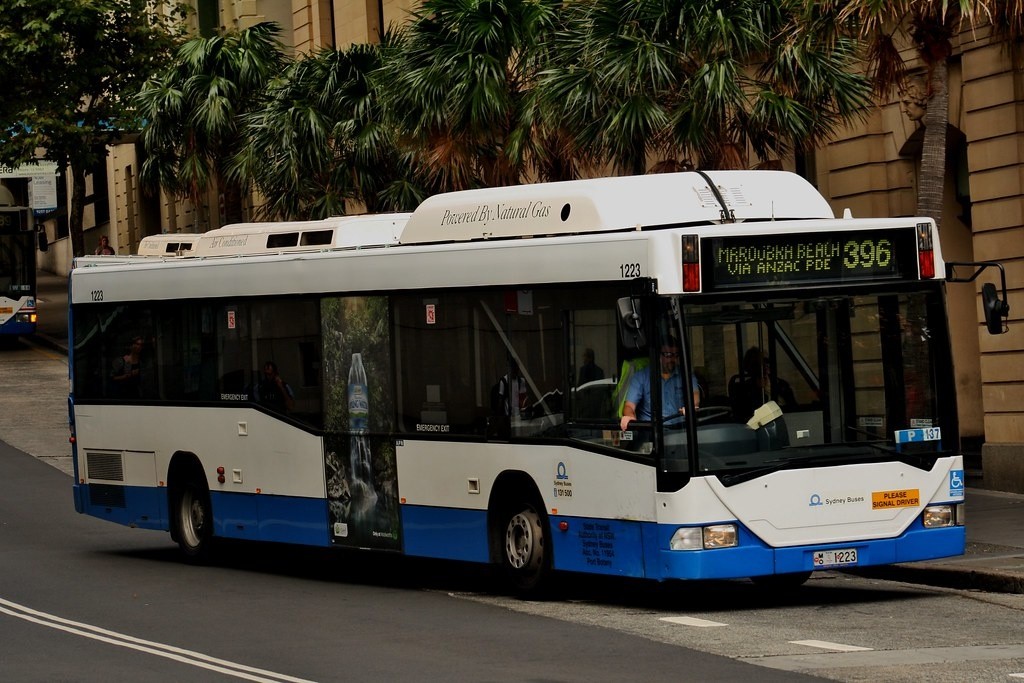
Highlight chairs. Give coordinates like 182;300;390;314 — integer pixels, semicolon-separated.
110;351;798;462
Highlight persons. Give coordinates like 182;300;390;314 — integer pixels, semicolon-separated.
499;360;531;439
578;348;607;420
110;334;157;404
94;236;115;255
621;334;701;432
252;362;296;414
749;356;779;413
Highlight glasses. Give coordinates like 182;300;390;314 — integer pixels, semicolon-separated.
661;352;680;358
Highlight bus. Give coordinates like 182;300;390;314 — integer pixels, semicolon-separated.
0;184;50;353
65;169;1011;602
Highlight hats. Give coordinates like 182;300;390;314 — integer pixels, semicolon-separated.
744;347;769;363
